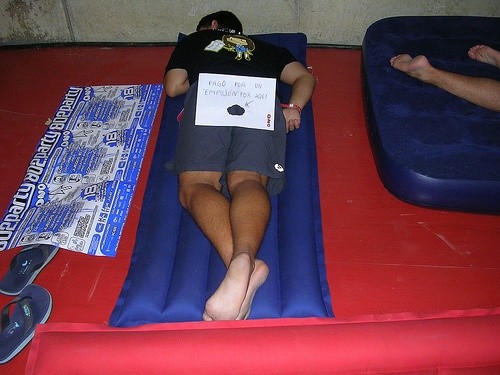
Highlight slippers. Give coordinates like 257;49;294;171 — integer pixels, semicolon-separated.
0;284;52;363
0;244;59;295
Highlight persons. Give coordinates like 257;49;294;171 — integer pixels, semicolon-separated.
390;44;500;112
164;10;316;321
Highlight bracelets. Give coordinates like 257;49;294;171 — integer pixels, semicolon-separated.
280;103;302;113
